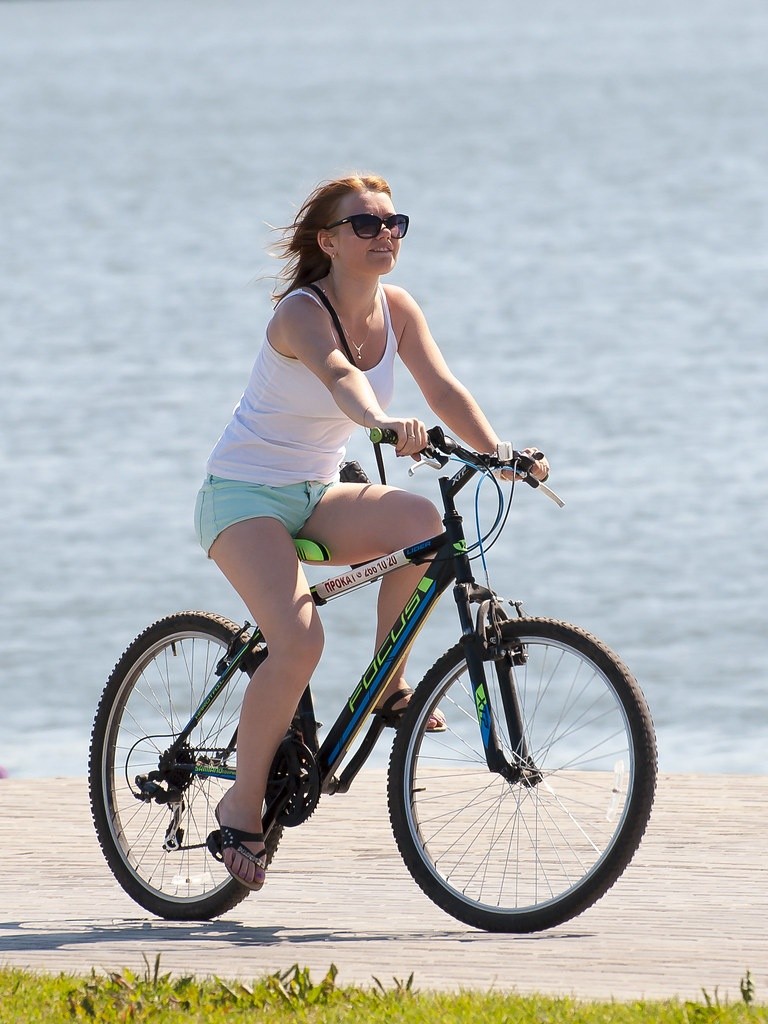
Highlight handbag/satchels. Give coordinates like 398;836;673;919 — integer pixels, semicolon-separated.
340;461;383;570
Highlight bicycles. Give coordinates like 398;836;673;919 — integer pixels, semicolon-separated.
84;419;659;937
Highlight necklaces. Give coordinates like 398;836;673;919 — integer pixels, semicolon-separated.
317;279;374;360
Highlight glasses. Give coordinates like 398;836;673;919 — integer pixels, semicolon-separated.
324;214;409;239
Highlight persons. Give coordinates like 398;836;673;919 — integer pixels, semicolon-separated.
192;176;550;892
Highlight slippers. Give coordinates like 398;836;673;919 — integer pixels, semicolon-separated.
370;687;446;732
216;805;266;891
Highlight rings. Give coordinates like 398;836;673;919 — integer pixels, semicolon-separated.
408;435;415;439
